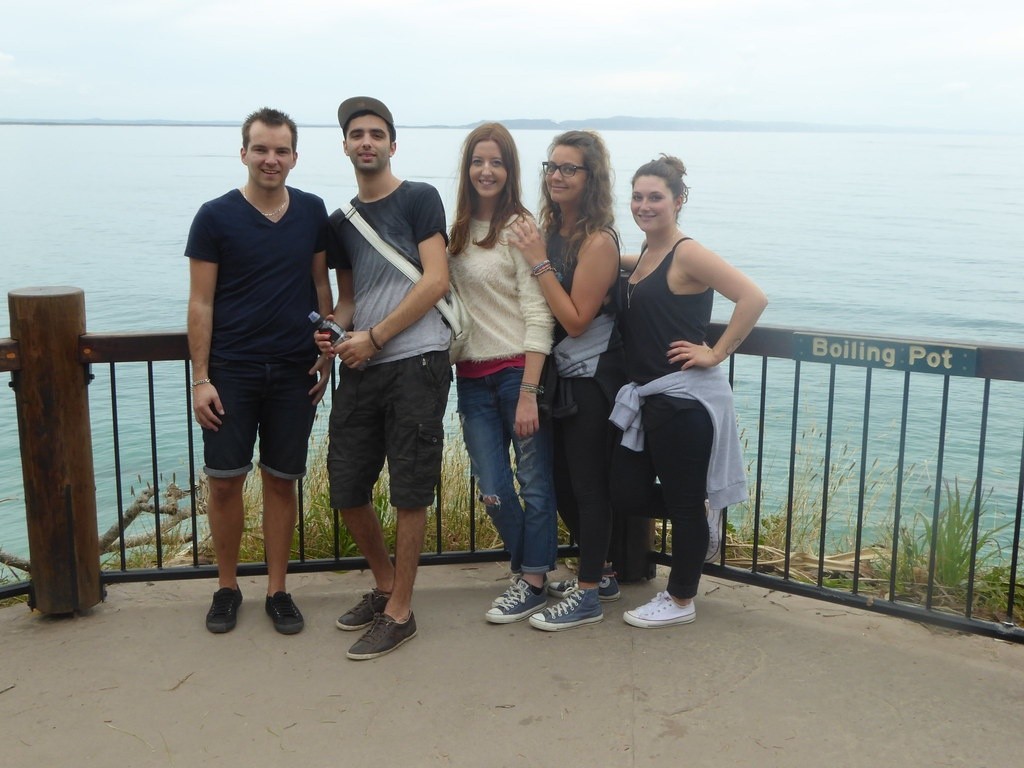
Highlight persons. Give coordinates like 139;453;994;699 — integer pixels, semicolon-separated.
618;151;768;629
313;96;454;659
506;131;626;634
446;122;559;624
185;107;334;635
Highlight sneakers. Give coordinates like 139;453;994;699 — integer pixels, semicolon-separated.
546;573;621;602
346;610;417;660
486;577;547;624
623;591;696;629
264;591;304;634
205;583;243;633
336;587;392;631
529;582;605;632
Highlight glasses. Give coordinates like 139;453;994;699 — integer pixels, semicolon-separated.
541;161;589;178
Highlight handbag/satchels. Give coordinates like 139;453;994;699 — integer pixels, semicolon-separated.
441;281;471;366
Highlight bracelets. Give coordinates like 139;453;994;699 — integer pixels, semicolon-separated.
192;378;210;386
531;260;557;276
520;382;544;395
369;327;383;351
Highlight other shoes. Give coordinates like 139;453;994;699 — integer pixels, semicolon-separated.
604;566;649;586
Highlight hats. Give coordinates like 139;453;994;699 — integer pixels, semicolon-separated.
337;95;394;127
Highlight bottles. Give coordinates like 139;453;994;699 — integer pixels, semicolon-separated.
308;310;370;372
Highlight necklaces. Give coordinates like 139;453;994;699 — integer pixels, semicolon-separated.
243;184;288;216
627;229;680;309
